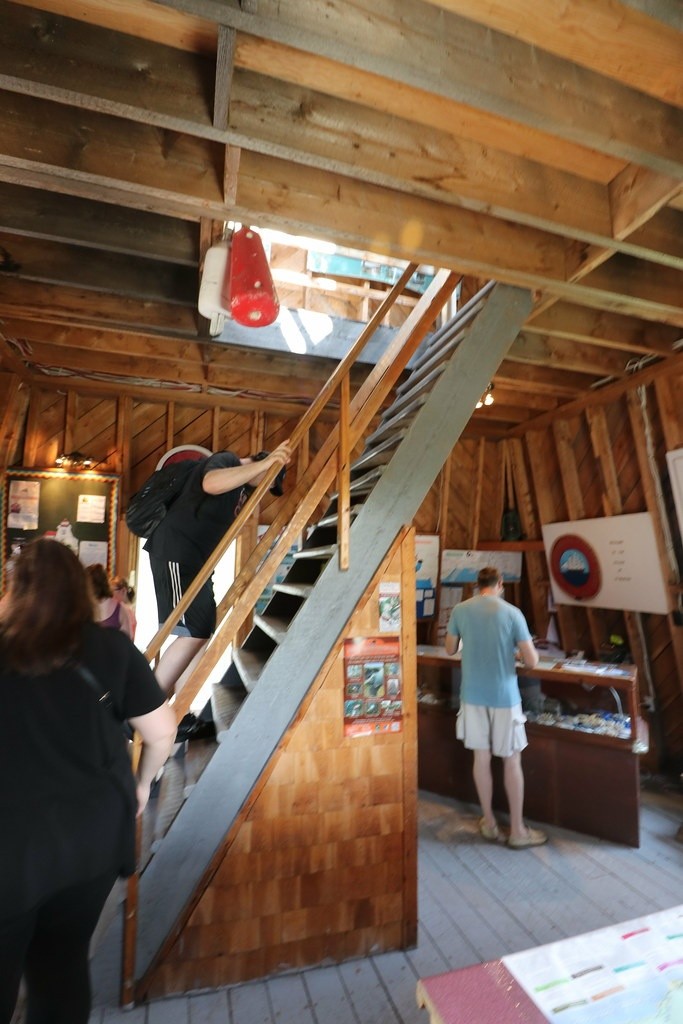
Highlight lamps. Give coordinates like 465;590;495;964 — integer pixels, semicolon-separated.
55;452;101;474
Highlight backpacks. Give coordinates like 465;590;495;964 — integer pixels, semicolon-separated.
126;459;200;538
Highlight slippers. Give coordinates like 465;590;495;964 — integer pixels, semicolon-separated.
478;815;499;842
506;824;548;849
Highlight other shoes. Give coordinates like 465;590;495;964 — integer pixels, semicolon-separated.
174;713;215;742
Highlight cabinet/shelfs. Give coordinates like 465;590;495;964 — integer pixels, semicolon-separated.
417;648;639;846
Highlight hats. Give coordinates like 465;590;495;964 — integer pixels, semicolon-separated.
256;452;286;496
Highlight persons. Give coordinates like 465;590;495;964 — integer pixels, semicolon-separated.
446;566;549;850
122;439;294;744
0;538;177;1024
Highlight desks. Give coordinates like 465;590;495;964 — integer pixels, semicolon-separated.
416;899;683;1023
